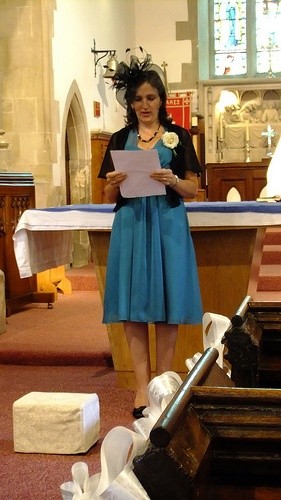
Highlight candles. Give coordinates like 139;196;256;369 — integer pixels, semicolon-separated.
219;113;224;139
245;120;249;141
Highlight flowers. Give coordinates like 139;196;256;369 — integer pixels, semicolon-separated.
160;131;183;156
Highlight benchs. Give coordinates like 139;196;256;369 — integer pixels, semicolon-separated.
220;295;281;388
129;348;281;500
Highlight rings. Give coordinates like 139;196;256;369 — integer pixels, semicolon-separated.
164;178;167;181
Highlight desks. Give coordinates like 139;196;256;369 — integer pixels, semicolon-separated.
0;184;54;318
11;201;281;373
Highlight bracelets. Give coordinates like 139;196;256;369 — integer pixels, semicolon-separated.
172;175;178;188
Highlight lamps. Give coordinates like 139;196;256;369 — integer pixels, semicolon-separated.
90;38;118;78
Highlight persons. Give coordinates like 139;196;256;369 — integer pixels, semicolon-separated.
97;70;203;419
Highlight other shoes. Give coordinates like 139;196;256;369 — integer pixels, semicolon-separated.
133;406;146;419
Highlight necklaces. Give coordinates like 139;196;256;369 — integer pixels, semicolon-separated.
137;122;162;142
